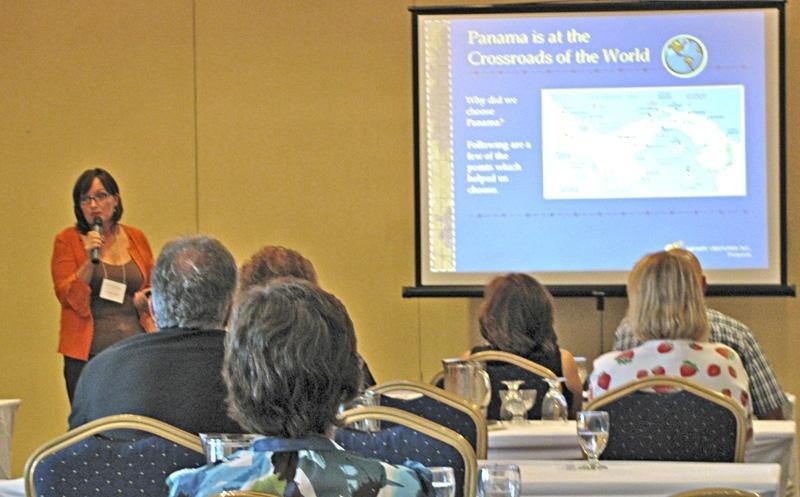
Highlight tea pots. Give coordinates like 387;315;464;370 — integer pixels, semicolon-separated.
439;356;493;424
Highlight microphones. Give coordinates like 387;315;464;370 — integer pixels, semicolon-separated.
88;218;104;265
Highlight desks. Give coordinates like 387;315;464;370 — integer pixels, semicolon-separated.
486;420;796;493
477;462;782;497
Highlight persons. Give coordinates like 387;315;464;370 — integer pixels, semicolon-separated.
230;245;375;399
590;251;753;447
67;235;252;437
163;278;438;497
612;248;789;419
51;168;160;410
462;274;582;421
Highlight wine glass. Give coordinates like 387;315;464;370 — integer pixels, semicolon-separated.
574;410;610;471
498;389;537;424
541;376;572;422
499;379;532;429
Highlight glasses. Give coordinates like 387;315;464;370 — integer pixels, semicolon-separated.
80;191;108;202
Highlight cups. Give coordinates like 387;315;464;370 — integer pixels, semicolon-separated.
571;355;590;388
476;462;523;496
198;431;242;467
427;465;458;497
340;389;383;431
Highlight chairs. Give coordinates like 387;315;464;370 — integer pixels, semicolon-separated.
330;405;477;497
581;375;748;463
429;351;566;423
364;379;487;458
22;414;218;497
